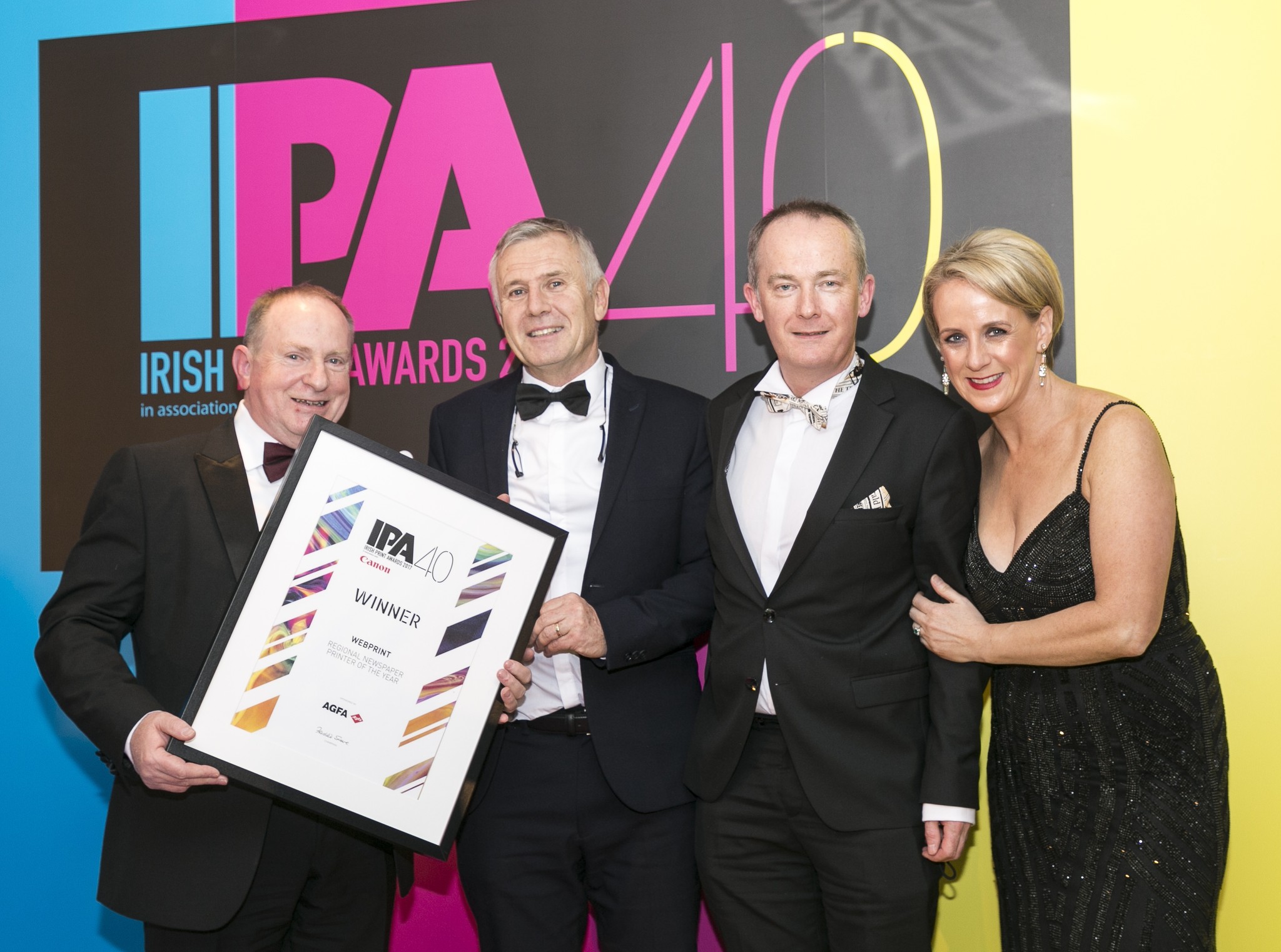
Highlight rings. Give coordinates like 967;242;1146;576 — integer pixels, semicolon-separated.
554;622;563;638
913;626;922;636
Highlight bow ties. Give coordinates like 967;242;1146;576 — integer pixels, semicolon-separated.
760;351;862;431
515;379;591;421
262;441;296;483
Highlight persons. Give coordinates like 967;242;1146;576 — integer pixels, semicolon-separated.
686;199;991;952
432;218;713;952
909;226;1231;952
35;282;534;952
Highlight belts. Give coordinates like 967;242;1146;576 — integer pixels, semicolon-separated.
506;711;588;732
